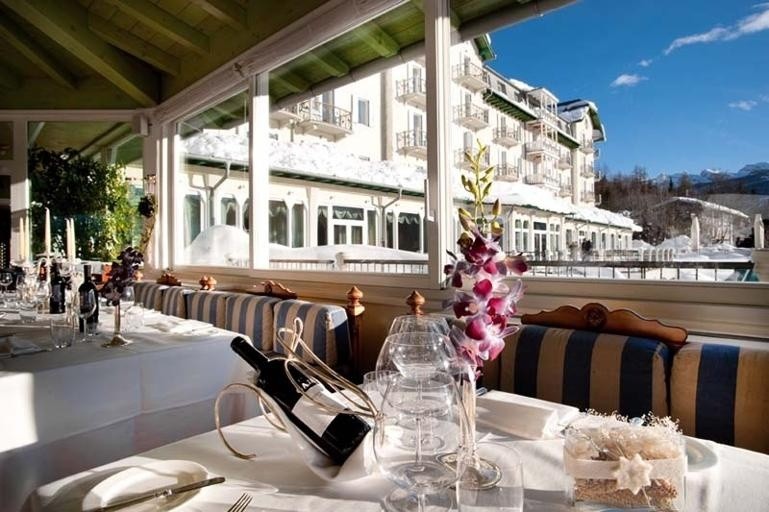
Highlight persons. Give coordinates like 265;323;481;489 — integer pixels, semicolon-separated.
566;237;593;263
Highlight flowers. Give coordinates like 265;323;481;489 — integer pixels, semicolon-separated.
436;130;534;376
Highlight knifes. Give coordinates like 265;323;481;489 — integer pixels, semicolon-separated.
89;476;226;511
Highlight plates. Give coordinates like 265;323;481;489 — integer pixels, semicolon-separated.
83;459;209;511
679;436;717;475
172;329;218;340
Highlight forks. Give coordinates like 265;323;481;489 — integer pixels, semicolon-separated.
228;493;254;512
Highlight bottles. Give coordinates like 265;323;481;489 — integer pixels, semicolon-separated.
230;337;372;467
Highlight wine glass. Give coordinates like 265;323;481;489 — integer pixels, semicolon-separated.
365;315;525;510
0;260;144;348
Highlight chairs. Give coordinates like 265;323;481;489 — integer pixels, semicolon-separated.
1;249;769;512
